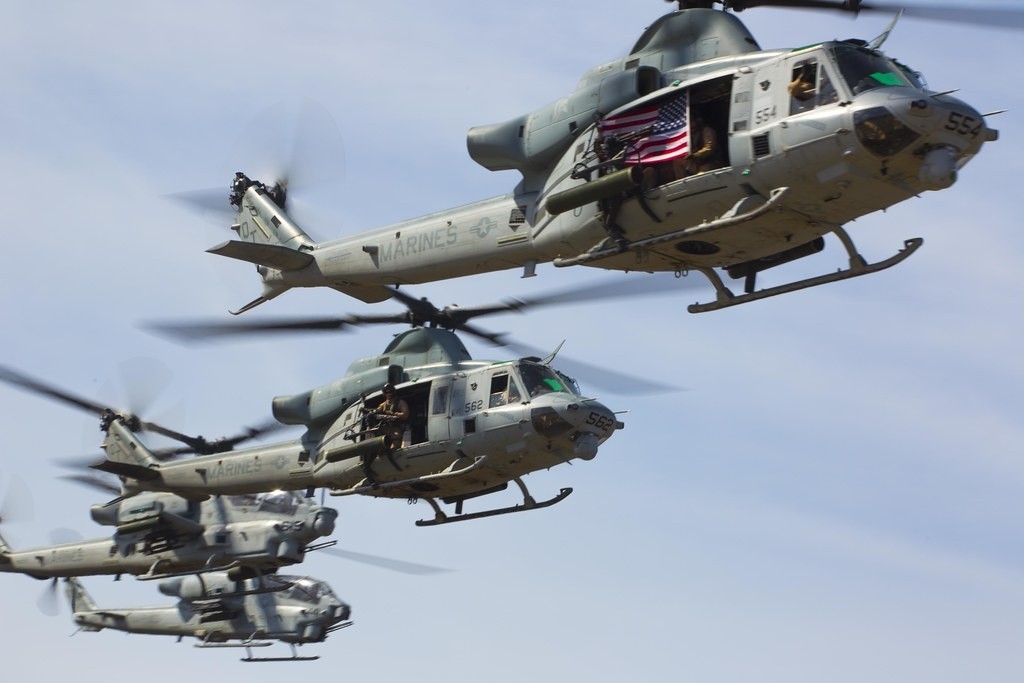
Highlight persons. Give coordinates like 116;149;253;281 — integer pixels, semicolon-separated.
372;383;410;453
685;115;724;175
789;60;830;109
594;127;630;246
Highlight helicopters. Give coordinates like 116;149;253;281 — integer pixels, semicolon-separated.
62;571;354;662
88;290;630;526
205;2;1007;315
0;371;338;581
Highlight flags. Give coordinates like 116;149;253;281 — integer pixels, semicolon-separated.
597;89;693;165
